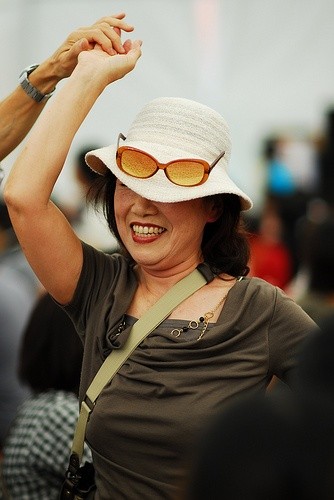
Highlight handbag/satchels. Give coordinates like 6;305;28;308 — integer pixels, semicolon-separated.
58;455;95;498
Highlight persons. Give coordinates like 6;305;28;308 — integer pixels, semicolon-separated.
116;283;231;342
0;13;134;163
0;25;319;500
0;293;92;500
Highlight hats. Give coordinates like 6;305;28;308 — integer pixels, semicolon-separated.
85;95;254;212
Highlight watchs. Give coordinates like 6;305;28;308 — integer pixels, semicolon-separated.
18;65;56;104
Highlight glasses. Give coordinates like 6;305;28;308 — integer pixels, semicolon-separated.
115;132;227;188
